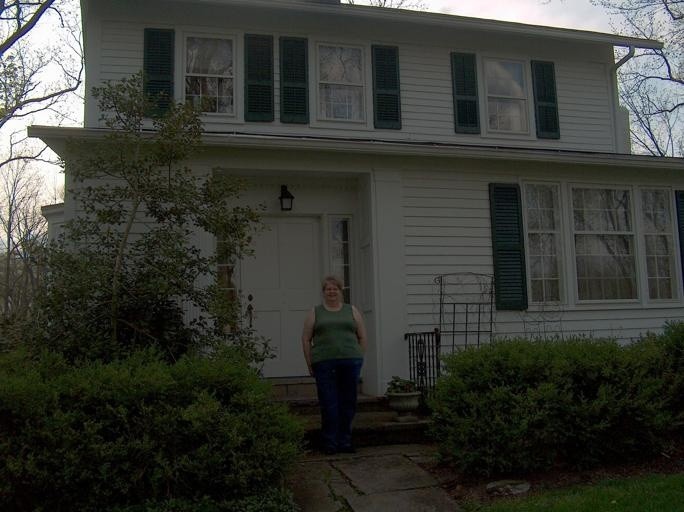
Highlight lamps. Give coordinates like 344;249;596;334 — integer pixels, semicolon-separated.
278;185;295;211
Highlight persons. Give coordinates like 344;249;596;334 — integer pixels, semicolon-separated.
302;274;369;458
